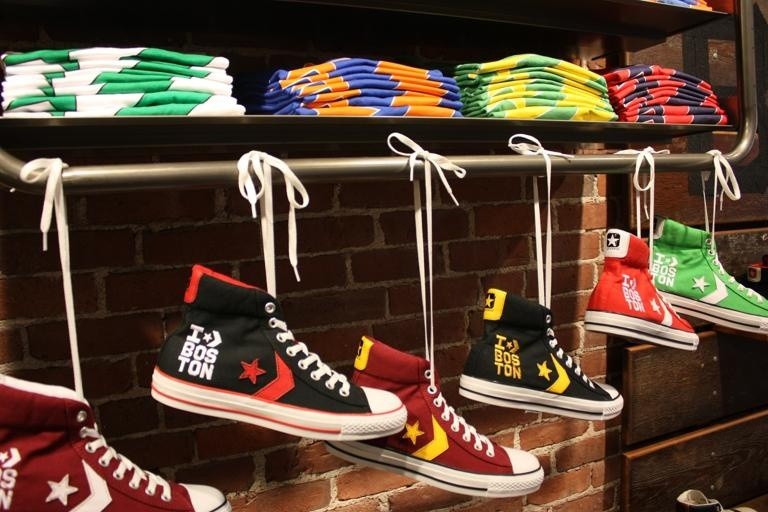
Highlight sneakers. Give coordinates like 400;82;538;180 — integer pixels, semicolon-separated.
648;218;768;335
675;488;758;512
149;264;409;442
583;228;700;352
323;335;545;498
458;287;624;421
0;372;232;512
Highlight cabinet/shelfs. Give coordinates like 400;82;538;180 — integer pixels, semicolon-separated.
0;1;735;147
